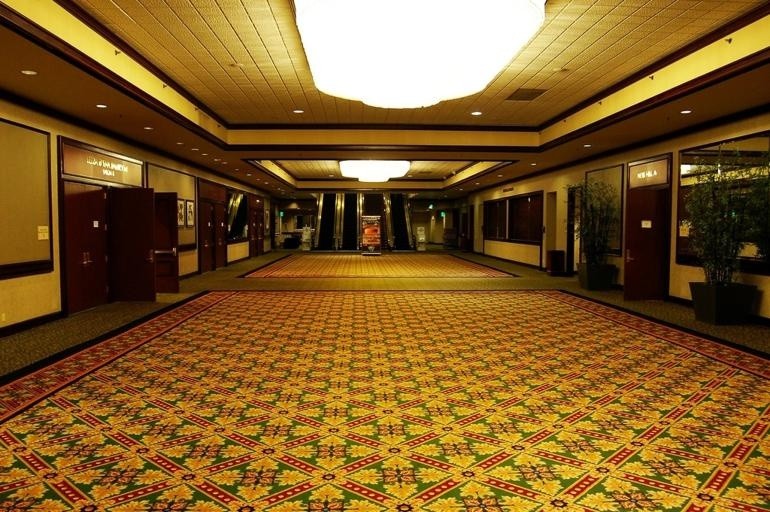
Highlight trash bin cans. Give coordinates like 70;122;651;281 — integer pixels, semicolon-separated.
545;248;566;277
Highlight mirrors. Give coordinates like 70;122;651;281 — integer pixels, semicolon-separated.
674;130;770;276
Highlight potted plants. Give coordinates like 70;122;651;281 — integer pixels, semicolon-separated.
689;141;756;327
573;181;616;293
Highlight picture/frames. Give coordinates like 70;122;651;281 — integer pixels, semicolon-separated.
177;198;195;228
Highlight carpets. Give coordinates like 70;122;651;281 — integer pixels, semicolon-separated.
1;250;770;509
